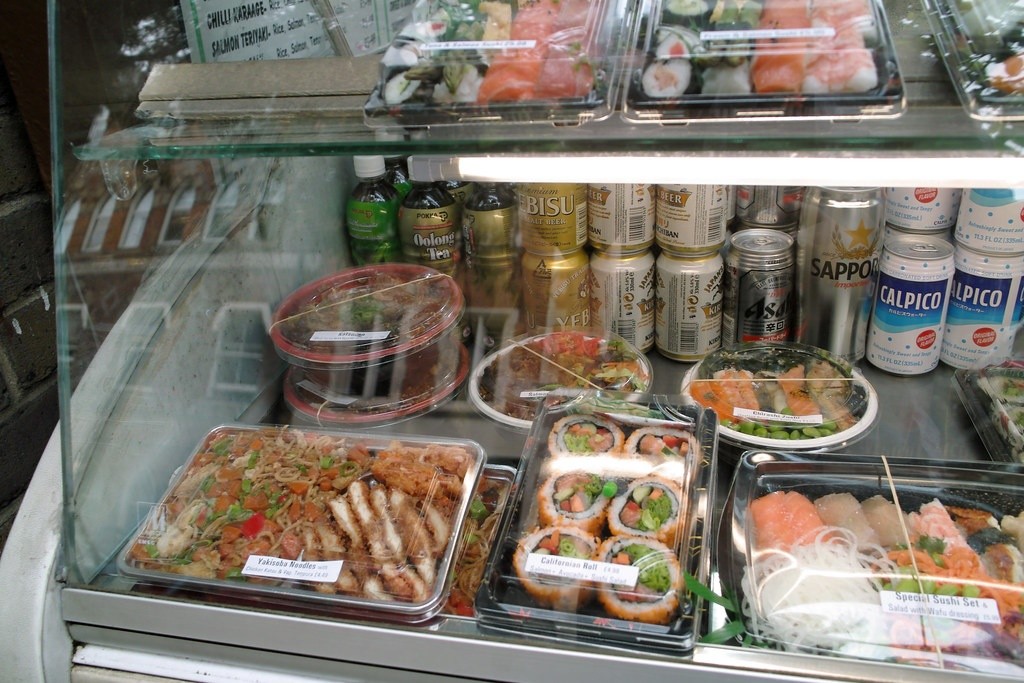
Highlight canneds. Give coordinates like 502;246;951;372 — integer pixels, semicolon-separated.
515;178;1024;375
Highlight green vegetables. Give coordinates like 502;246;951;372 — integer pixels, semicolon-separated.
870;534;980;598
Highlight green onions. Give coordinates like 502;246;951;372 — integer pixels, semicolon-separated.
141;438;359;585
338;300;387;326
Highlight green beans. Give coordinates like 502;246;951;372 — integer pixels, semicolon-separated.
720;408;837;439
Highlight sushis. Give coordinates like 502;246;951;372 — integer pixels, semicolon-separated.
380;0;1024;105
511;415;702;625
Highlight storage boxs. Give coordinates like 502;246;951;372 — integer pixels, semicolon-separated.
106;0;1024;683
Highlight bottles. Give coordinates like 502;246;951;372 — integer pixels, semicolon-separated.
437;181;476;211
398;183;469;345
376;135;410;199
462;182;524;337
349;155;399;267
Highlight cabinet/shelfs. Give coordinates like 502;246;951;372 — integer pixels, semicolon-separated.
0;0;1024;683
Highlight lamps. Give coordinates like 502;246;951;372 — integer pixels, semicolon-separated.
408;151;1024;189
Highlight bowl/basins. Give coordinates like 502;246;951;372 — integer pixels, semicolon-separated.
681;361;879;453
271;263;467;385
469;337;649;434
282;337;467;428
